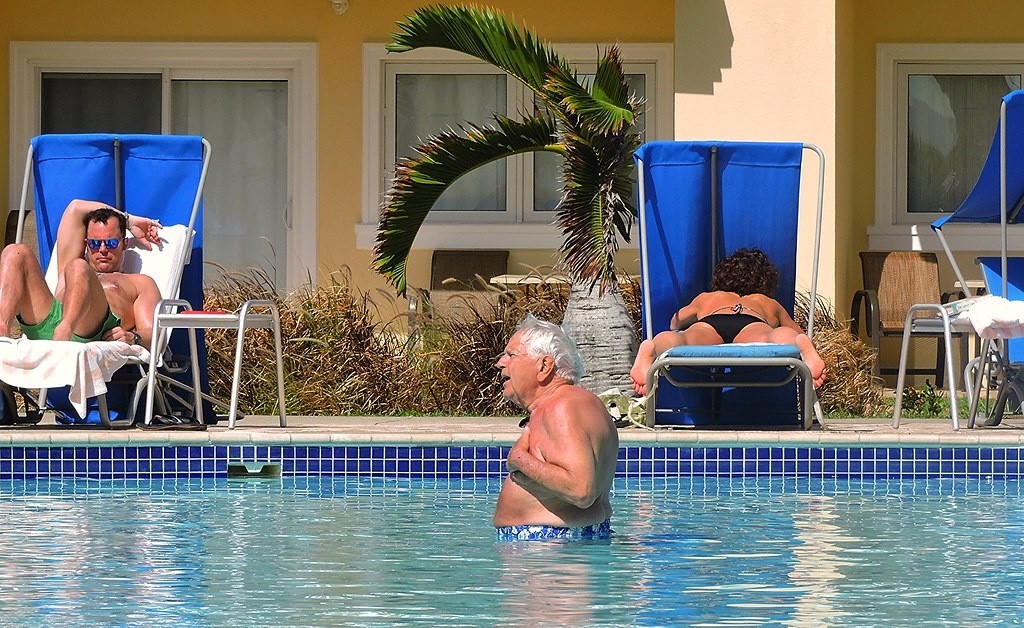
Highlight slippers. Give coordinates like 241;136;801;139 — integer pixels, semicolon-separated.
136;415;207;431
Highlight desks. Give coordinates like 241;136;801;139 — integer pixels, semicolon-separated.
490;274;641;302
893;304;1004;430
145;298;287;429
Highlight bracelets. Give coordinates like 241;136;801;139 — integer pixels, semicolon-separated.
125;212;129;228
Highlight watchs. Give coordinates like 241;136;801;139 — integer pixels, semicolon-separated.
132;331;142;345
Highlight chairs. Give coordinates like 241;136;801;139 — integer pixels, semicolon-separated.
930;91;1024;426
1;134;219;428
851;251;967;388
409;251;510;349
631;140;825;431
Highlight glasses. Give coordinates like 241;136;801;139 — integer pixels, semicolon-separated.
85;238;123;250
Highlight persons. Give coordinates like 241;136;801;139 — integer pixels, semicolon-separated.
629;248;826;395
493;315;618;542
0;199;163;351
